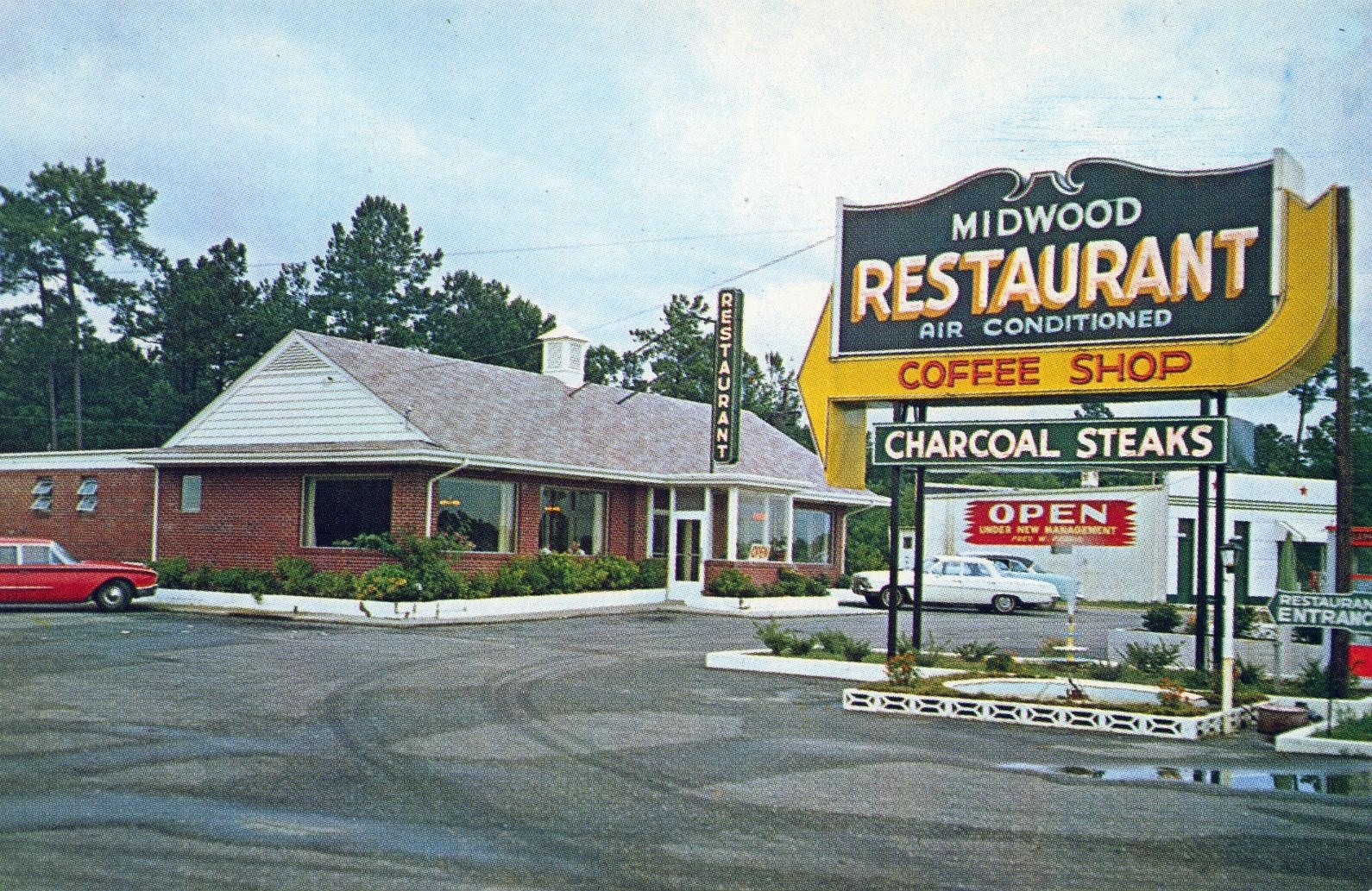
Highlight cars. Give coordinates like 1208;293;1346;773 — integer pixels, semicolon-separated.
947;552;1085;603
849;555;1060;614
1;537;160;613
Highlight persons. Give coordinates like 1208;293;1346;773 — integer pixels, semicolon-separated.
538;539;586;556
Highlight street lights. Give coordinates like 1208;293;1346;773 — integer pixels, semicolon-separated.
1219;535;1262;737
